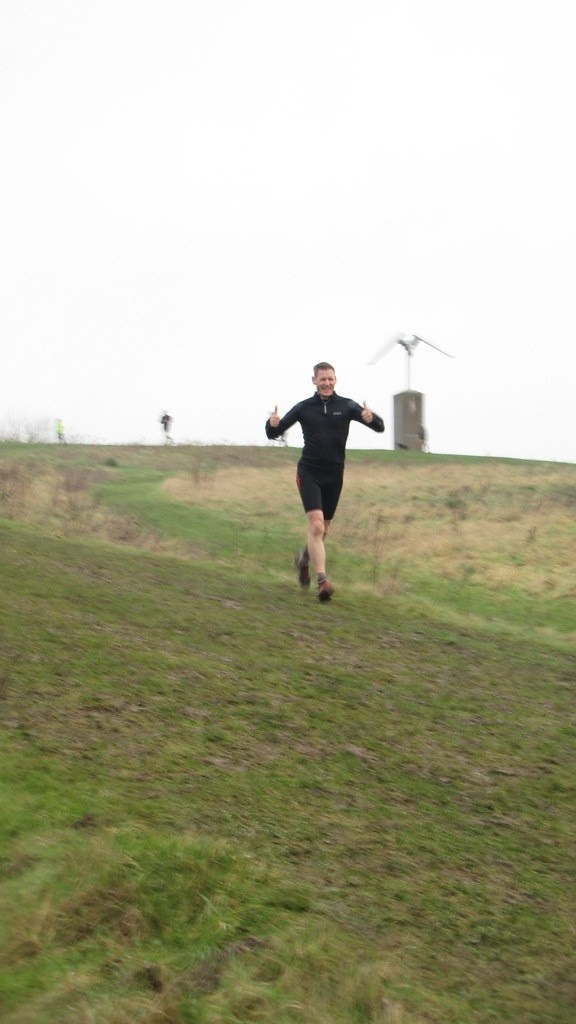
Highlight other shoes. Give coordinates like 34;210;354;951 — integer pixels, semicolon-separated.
318;579;333;600
296;562;311;588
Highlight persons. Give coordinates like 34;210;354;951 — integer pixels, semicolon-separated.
161;410;173;445
265;361;385;603
416;423;429;452
55;419;67;444
